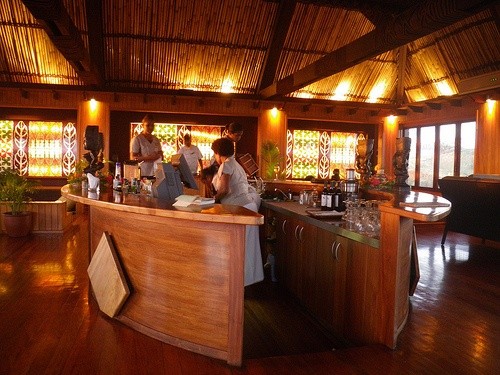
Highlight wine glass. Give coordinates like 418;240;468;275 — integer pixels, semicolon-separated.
341;198;381;237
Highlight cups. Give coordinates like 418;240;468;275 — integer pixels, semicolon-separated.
300;190;318;207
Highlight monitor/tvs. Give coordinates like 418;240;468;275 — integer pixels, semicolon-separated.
153;154;199;201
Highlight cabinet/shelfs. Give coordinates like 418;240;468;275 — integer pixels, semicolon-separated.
288;219;317;307
314;227;369;343
274;215;291;289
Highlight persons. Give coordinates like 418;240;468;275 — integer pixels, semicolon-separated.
212;121;243;164
179;134;203;176
212;138;264;287
132;116;163;179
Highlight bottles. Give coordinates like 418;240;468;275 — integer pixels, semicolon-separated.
115;162;152;195
320;181;343;212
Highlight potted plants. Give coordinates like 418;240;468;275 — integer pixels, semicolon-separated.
0;164;35;240
